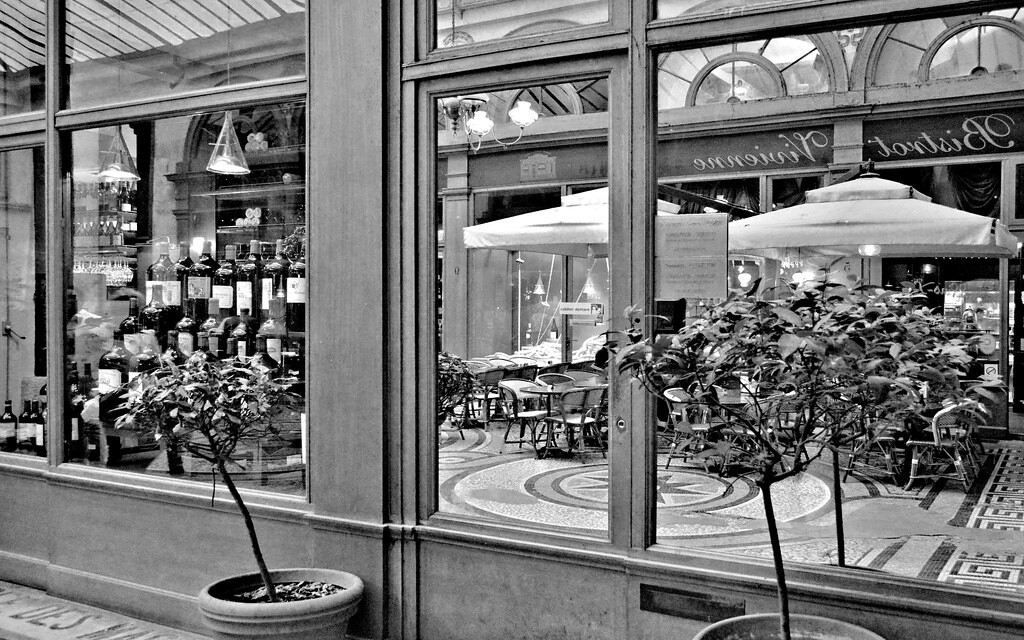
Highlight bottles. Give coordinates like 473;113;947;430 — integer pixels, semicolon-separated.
99;240;307;421
0;384;48;457
74;181;137;212
64;358;99;460
73;215;124;246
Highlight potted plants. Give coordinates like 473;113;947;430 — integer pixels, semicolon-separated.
110;353;366;639
789;278;1009;574
599;275;921;640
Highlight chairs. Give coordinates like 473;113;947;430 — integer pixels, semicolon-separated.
662;376;982;494
462;354;608;464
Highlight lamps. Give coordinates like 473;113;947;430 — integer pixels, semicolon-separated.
206;0;251;176
96;0;142;185
433;0;545;152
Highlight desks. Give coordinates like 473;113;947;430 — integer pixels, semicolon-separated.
708;389;785;405
565;379;608;387
519;384;573;459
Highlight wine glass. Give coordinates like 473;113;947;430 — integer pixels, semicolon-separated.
73;256;133;287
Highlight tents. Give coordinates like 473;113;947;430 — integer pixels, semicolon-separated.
462;173;1018;442
